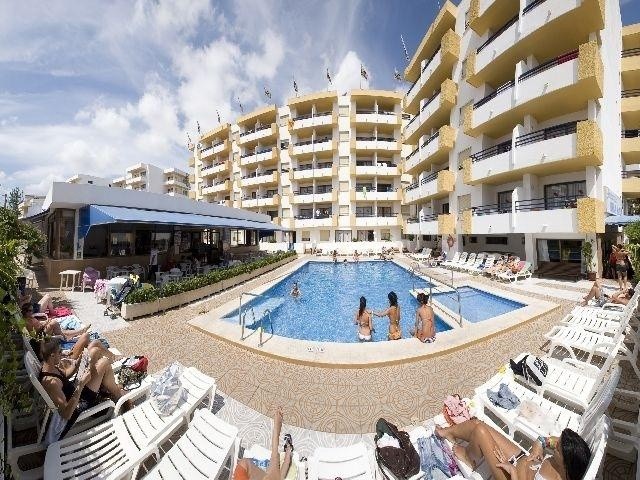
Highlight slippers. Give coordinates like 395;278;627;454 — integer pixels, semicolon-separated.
282;434;294;452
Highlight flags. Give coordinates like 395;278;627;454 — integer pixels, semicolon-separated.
361;64;368;80
394;70;401;80
238;98;245;112
293;81;299;93
327;71;333;85
216;110;227;123
264;88;271;98
196;121;201;134
187;132;191;142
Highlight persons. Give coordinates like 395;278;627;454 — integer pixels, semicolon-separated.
406;293;437;343
373;290;402;340
232;404;294;480
583;281;636;306
609;252;617;279
353;296;373;342
435;418;592;480
483;253;522;276
316;208;321;219
3;293;134;420
611;243;633;291
290;282;301;298
310;246;395;263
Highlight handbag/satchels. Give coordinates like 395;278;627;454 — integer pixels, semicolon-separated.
118;358;148;391
510;353;548;387
371;419;420;480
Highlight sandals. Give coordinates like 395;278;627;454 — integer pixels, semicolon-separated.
487;385;520;410
